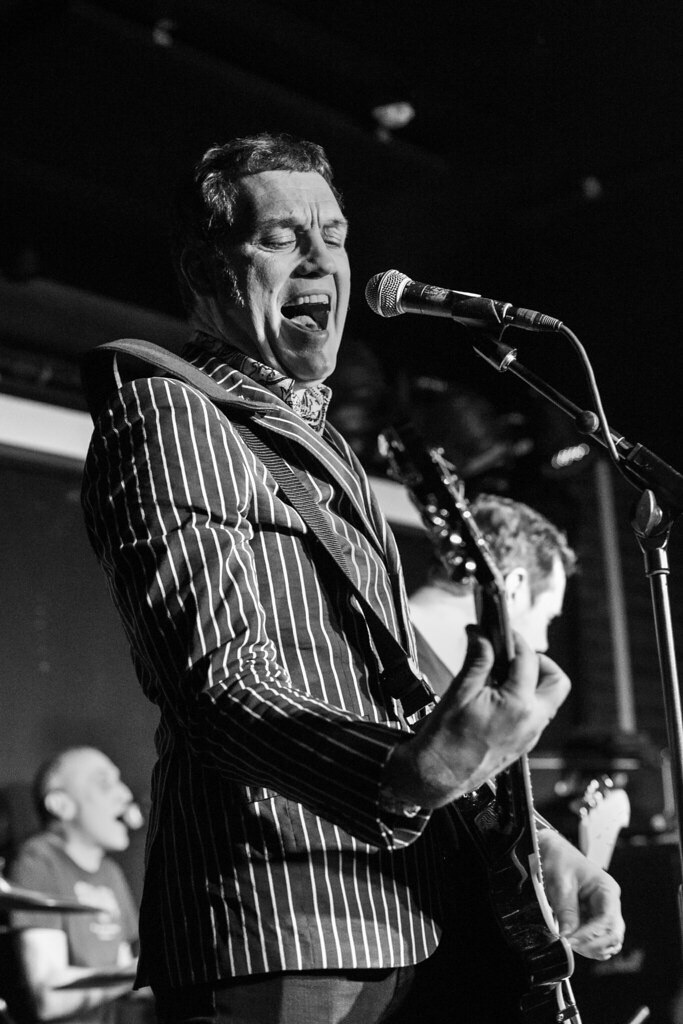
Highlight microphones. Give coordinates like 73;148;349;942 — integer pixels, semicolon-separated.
365;269;563;333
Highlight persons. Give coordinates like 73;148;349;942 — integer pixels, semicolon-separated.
10;746;164;1023
82;130;630;1024
408;493;582;712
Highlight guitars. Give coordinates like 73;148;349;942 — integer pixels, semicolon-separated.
379;431;583;1024
